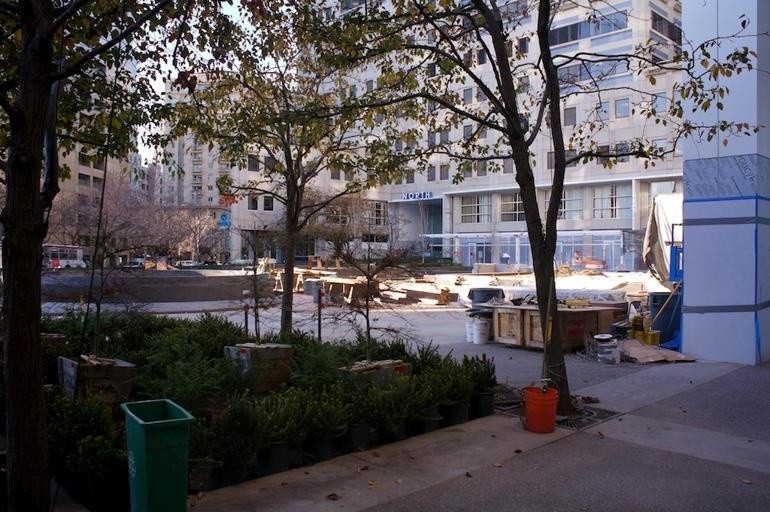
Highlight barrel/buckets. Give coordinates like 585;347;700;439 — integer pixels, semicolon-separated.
463;320;474;342
522;386;560;432
304;280;318;294
472;321;490;344
596;337;621;365
312;285;323;304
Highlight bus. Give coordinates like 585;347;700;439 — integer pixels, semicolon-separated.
176;260;198;267
42;243;86;269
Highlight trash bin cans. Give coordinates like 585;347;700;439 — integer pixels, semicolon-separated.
500;257;509;264
648;292;682;345
468;288;506;318
120;398;196;512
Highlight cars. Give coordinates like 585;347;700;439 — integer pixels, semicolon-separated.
123;260;144;268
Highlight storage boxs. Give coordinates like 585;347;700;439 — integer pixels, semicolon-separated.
493;311;523;346
525;310;613;353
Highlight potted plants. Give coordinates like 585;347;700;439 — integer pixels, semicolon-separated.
188;357;393;492
367;352;495;445
